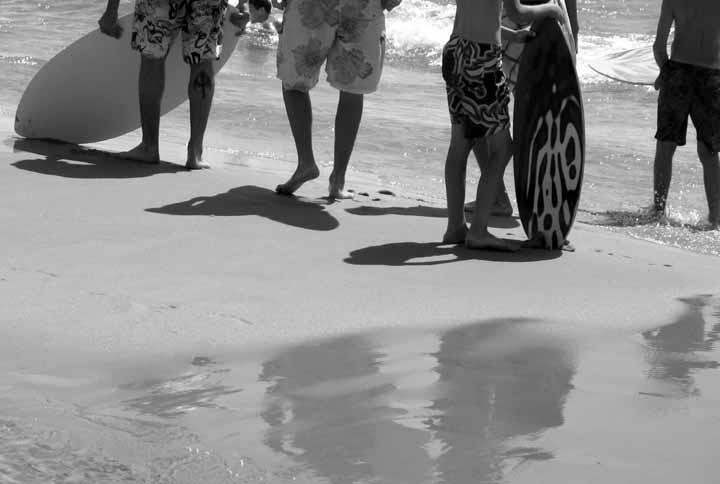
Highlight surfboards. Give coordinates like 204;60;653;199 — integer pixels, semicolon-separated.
512;1;585;250
588;42;677;83
13;3;244;144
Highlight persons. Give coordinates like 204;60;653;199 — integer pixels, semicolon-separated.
442;0;566;252
464;0;578;215
653;0;720;230
249;0;282;34
100;0;249;168
273;0;402;200
